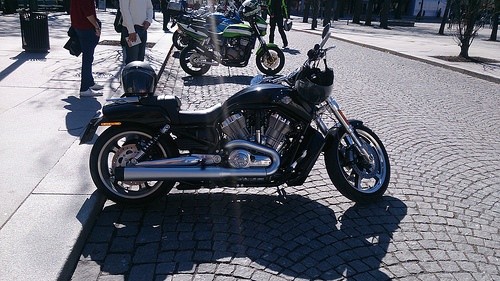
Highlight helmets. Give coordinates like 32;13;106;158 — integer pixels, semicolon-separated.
242;0;262;17
122;61;158;97
284;20;292;31
295;66;335;104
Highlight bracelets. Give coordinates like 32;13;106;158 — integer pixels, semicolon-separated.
95;26;101;30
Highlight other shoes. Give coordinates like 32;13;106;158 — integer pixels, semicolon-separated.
89;83;104;90
79;88;104;97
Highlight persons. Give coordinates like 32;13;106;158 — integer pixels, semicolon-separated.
267;0;289;48
161;0;171;33
118;0;154;66
69;0;104;97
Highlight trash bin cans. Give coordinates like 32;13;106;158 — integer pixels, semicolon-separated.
19;11;50;53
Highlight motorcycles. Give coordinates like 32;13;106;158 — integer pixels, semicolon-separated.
167;0;284;77
79;23;390;206
